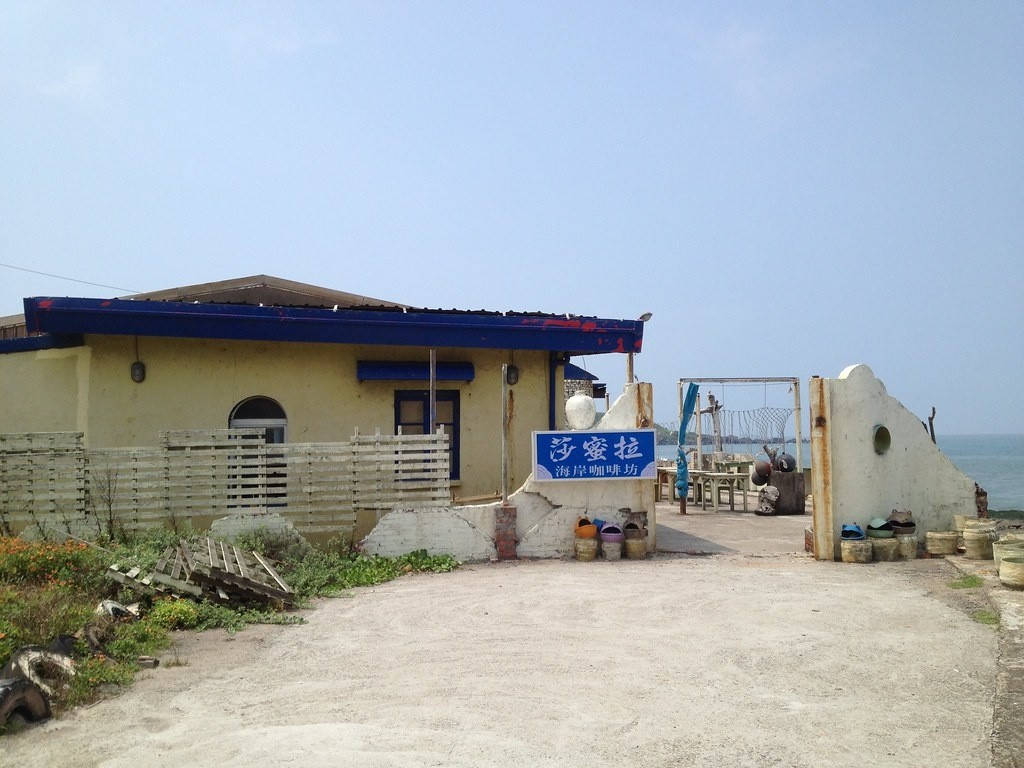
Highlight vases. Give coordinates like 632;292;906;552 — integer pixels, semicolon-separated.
624;538;647;560
601;542;622;562
574;538;598;562
842;514;1024;589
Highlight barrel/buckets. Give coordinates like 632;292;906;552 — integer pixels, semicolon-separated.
926;521;1024;590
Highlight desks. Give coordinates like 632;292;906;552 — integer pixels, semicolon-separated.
657;467;750;513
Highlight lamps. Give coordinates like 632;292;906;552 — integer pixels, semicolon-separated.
506;363;517;384
130;361;145;383
